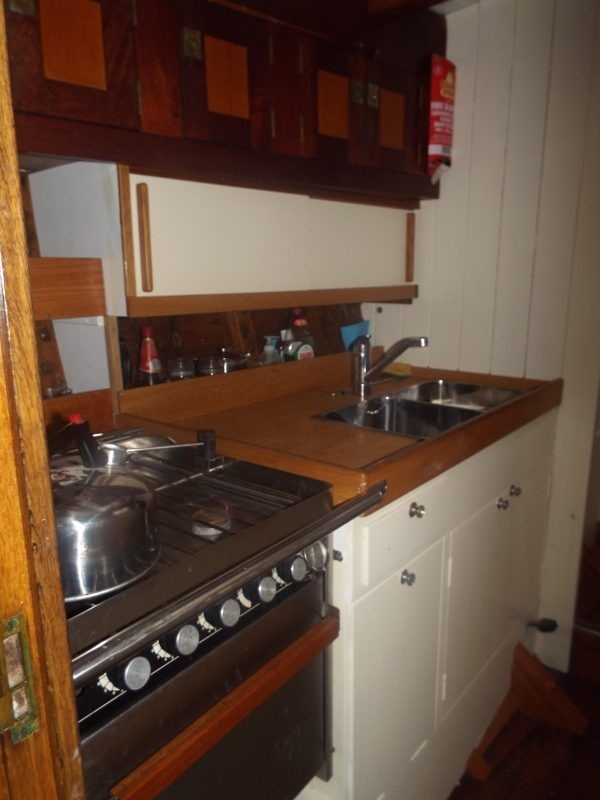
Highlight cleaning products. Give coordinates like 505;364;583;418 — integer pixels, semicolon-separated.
262;334;280;364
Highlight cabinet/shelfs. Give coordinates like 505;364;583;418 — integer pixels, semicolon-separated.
27;161;419;317
287;407;558;799
3;0;448;211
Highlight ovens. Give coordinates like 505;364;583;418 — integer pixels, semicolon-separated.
67;481;389;800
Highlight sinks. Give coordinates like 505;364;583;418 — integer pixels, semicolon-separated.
389;377;528;413
310;396;482;442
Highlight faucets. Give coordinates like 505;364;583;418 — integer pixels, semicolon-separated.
348;333;429;397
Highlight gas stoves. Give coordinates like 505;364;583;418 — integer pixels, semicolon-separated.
48;428;337;666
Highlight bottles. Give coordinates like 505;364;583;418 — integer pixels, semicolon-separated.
140;327;165;386
260;308;317;367
38;330;63;399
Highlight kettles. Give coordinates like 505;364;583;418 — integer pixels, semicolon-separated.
48;415;163;603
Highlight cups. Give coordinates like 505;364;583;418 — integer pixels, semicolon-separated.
169;347;259;383
